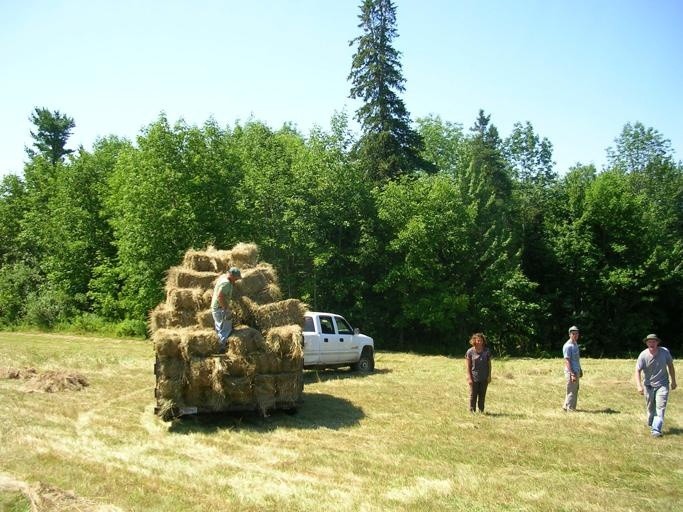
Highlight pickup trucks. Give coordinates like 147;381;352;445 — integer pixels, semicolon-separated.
302;311;375;375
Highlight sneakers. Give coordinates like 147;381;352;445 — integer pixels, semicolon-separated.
654;433;663;437
564;407;576;411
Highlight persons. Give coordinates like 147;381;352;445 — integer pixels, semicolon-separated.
464;332;491;416
211;267;242;353
562;325;583;413
635;333;677;438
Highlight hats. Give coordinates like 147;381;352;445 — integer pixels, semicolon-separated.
643;334;661;343
228;268;242;279
569;326;579;332
